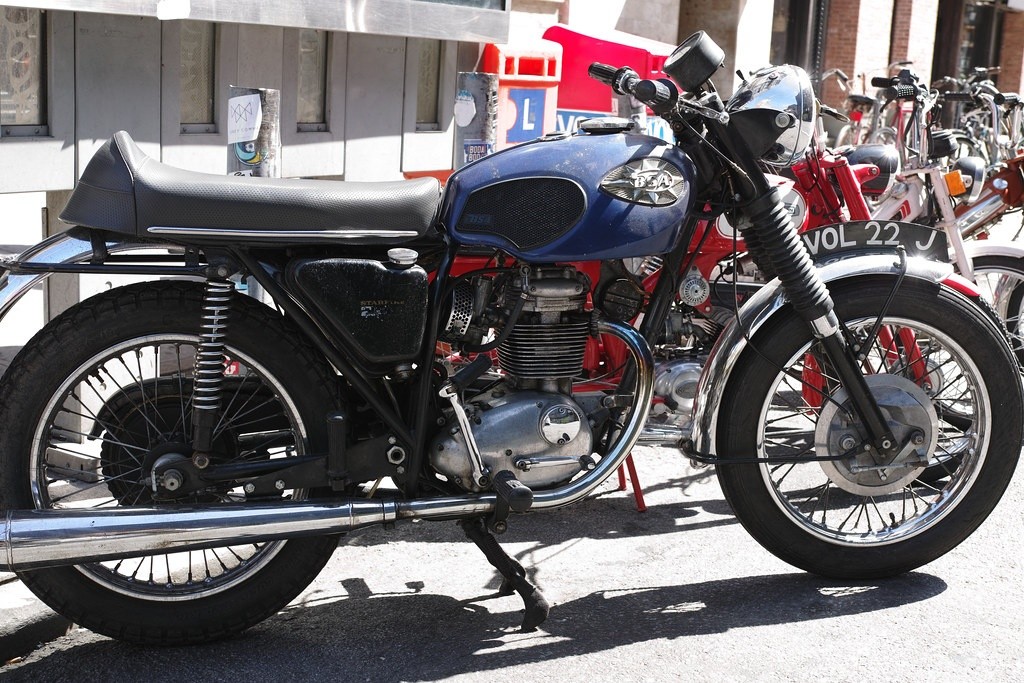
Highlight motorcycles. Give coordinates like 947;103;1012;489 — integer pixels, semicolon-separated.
0;32;1024;651
416;62;1023;412
